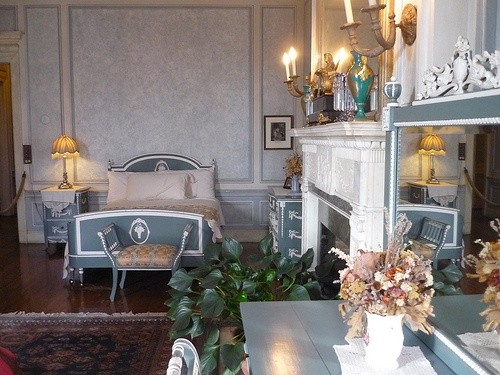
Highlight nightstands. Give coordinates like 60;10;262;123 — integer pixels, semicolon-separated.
267;191;302;269
38;186;91;252
407;181;458;208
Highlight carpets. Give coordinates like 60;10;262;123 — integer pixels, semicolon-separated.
0;312;220;375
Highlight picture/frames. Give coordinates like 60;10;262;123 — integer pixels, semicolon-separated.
264;114;293;149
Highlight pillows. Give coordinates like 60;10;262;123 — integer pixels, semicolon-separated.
126;174;194;199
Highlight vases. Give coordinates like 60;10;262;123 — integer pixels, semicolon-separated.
292;176;299;191
364;308;406;371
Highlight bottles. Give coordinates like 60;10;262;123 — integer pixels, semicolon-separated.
346;49;374;120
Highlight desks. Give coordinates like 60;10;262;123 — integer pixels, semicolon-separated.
240;294;500;375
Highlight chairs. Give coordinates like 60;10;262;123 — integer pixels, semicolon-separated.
165;338;202;375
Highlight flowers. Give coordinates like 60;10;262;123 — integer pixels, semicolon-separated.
466;216;500;332
328;205;437;338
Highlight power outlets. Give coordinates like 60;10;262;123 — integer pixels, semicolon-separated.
24;164;29;175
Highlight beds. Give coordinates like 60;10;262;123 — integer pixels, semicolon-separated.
397;199;465;265
62;152;226;287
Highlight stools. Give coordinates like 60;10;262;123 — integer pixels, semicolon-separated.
97;222;193;302
408;217;450;268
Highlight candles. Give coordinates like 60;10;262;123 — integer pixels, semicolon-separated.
282;0;377;81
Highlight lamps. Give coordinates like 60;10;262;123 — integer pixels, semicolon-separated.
418;132;445;184
51;135;79;189
